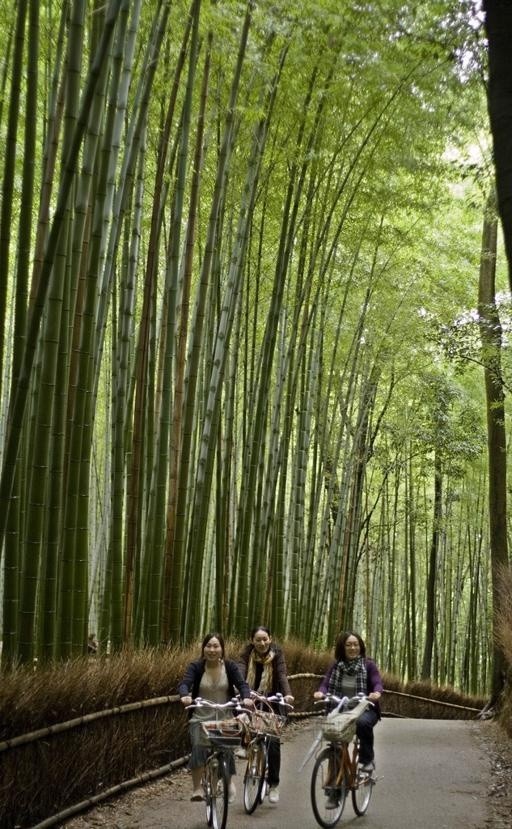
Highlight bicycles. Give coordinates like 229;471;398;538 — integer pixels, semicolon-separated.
234;690;295;814
180;696;252;828
309;691;386;828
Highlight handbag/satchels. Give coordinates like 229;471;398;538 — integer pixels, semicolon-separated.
254;710;281;735
323;712;356;742
200;717;241;746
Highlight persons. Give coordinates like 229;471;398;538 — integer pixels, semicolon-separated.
314;632;384;810
178;631;253;801
237;625;295;804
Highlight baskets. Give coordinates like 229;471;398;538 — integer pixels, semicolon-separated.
252;710;287;736
198;722;243;749
321;717;356;743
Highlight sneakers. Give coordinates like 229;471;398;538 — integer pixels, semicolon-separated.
268;785;280;803
324;799;339;809
360;762;375;777
192;790;203;800
236;748;246;758
